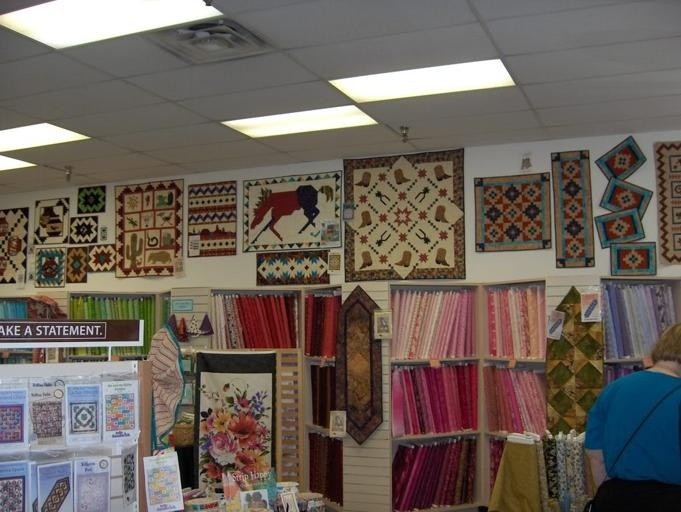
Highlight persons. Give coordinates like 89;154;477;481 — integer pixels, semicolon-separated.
583;321;681;511
